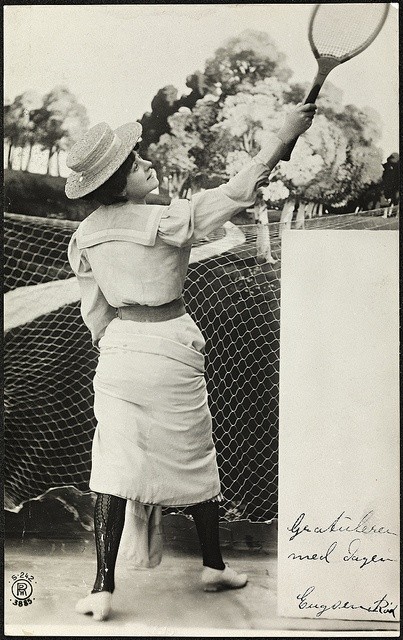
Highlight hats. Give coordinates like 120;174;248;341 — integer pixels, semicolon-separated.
64;122;143;200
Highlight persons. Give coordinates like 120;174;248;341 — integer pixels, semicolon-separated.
64;104;317;622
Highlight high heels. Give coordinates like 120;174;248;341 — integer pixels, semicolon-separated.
75;591;111;620
200;563;248;591
